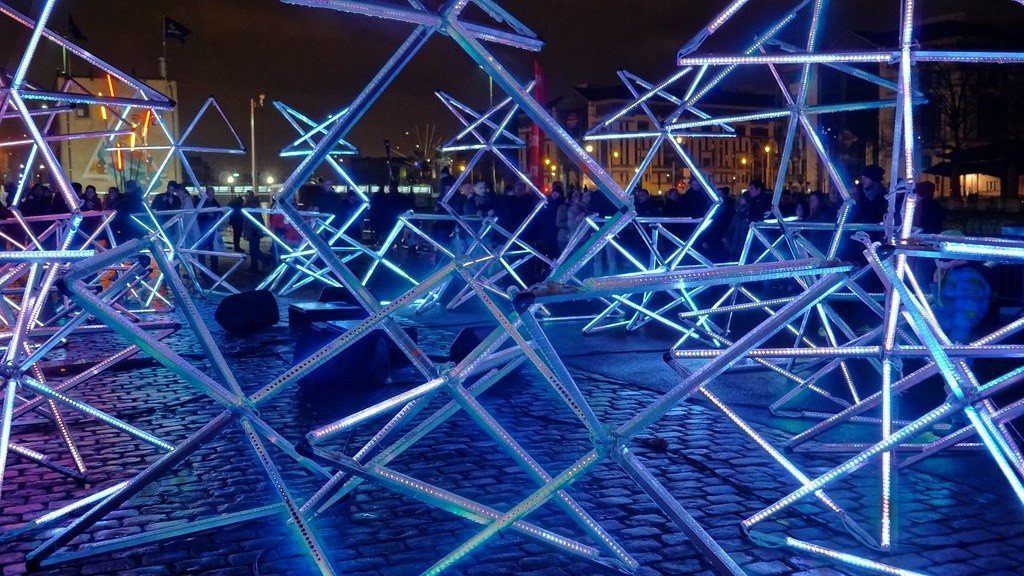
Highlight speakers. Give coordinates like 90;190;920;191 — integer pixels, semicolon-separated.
216;289;280;334
446;322;529;379
320;285;380;315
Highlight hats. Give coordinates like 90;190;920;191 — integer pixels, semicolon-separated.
551;184;562;194
916;181;935;197
862;164;885;183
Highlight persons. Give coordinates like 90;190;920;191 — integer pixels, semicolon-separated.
0;163;1024;439
